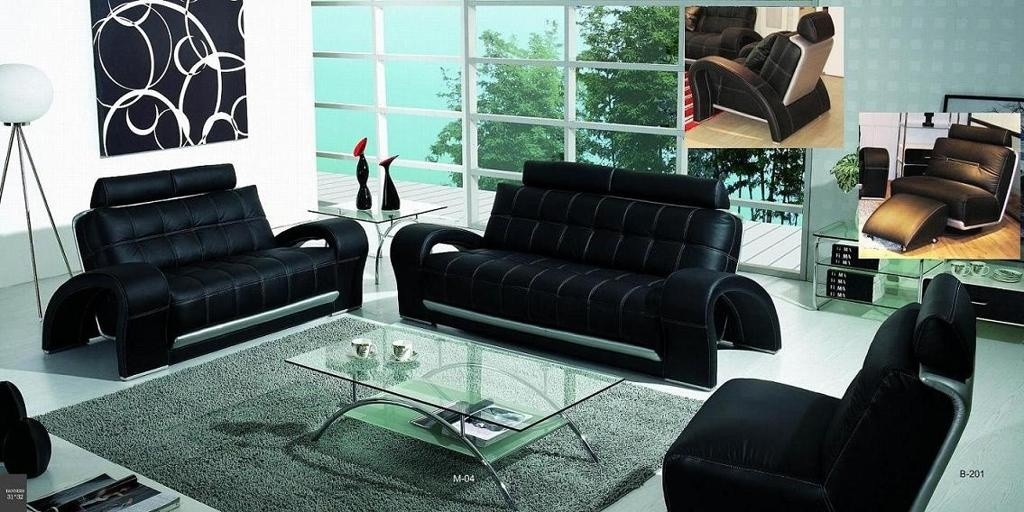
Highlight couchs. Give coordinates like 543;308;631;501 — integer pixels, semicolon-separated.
390;162;782;391
41;163;369;383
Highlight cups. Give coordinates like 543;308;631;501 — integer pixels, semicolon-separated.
394;340;413;361
351;337;374;359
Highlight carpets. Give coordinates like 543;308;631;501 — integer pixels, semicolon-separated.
31;316;704;509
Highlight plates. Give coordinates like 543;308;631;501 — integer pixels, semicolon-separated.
345;344;377;357
384;352;420;363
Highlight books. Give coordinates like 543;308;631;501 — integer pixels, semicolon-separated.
26;471;181;512
831;240;890;270
409;398;533;448
826;267;886;302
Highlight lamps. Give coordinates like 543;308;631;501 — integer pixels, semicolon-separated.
0;63;76;330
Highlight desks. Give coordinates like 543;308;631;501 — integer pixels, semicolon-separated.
814;218;944;310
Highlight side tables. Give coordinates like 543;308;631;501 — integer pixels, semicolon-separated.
308;197;446;287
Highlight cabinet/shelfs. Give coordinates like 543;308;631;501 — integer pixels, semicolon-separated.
896;143;934;178
916;259;1024;328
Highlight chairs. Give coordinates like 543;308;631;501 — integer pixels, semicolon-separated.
684;7;835;143
860;122;1019;252
661;273;975;512
